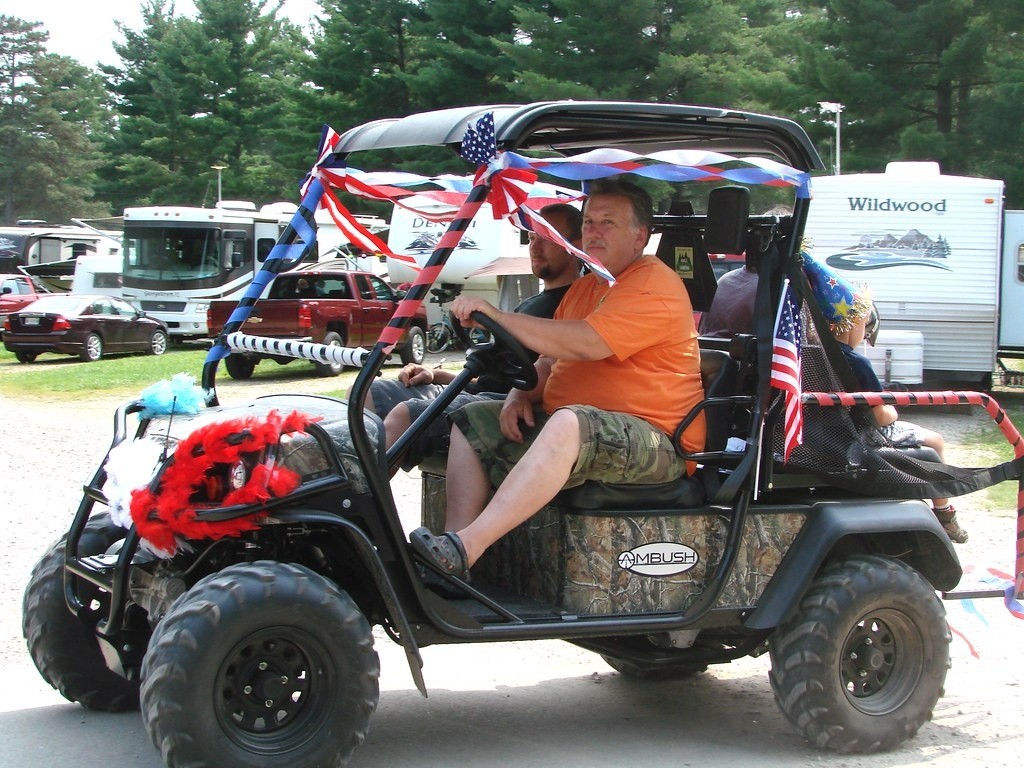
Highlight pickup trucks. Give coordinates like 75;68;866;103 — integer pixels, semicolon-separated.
205;267;429;378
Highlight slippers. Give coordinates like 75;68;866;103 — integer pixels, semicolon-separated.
409;526;471;593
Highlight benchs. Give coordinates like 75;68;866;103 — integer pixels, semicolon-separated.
420;349;731;616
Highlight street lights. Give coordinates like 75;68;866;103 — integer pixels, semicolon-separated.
816;100;845;178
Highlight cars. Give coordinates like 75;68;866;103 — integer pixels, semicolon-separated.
1;275;40;341
2;294;170;364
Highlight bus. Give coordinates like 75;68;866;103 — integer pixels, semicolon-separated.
120;200;389;347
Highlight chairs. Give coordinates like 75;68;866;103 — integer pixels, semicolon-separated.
298;281;317;296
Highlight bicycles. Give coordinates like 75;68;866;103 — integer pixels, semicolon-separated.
424;303;490;355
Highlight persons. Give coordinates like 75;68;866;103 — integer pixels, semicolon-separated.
409;179;706;583
698;205;970;542
346;203;586;482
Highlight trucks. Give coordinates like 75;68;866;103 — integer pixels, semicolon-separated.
999;209;1024;365
388;182;677;332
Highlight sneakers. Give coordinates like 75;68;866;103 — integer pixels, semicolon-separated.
932;505;968;543
